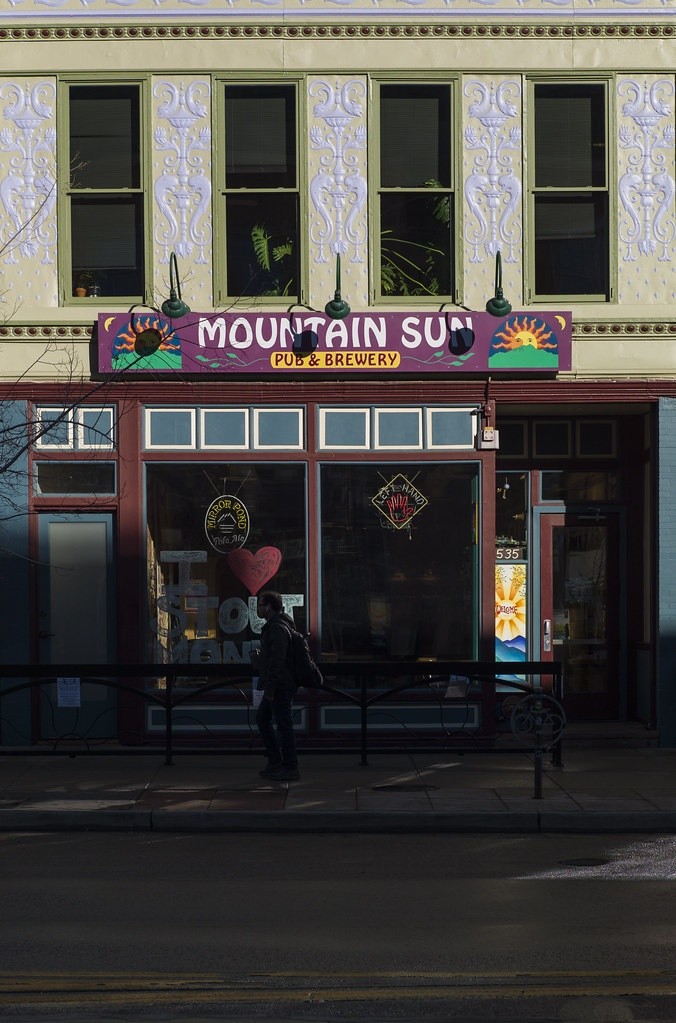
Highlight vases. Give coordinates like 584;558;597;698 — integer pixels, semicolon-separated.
76;288;86;297
89;286;101;298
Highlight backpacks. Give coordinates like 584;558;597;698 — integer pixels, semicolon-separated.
272;619;312;683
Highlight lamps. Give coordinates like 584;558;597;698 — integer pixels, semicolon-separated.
325;253;350;320
485;250;512;317
161;252;188;319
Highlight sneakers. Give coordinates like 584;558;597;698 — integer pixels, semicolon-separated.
259;763;301;781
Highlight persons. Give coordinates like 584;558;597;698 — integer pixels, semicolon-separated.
253;591;298;779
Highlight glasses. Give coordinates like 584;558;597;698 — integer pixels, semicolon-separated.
258;603;266;608
